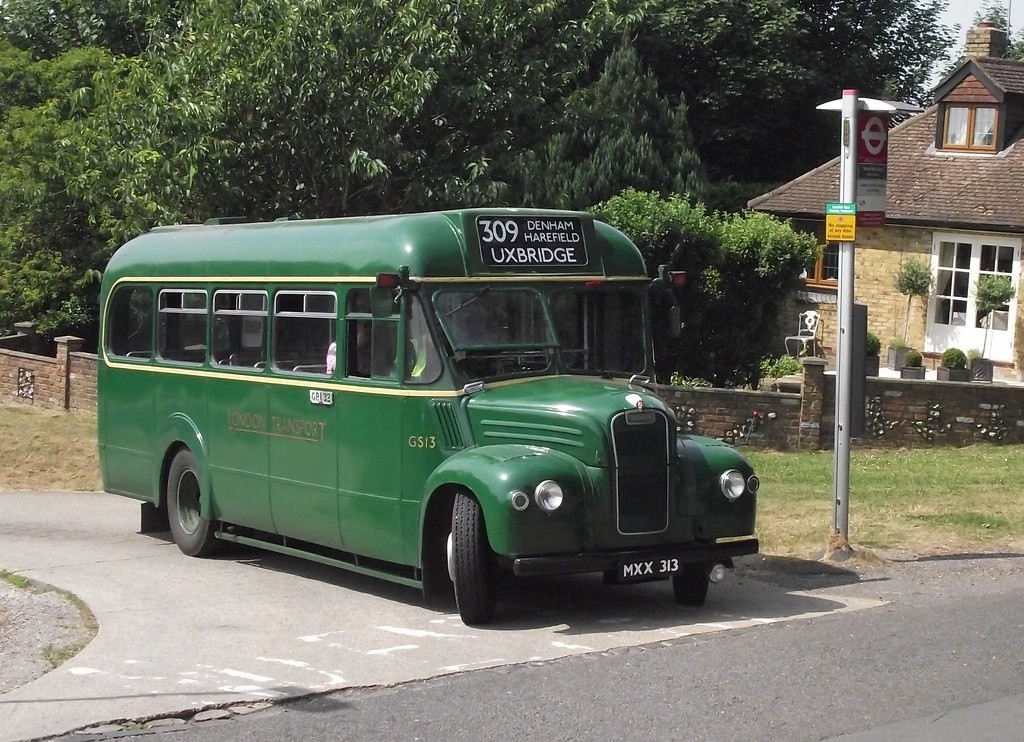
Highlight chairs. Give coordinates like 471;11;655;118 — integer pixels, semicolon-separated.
785;309;820;360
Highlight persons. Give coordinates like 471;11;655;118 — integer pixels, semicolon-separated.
324;320;372;376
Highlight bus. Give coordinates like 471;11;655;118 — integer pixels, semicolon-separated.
97;207;765;623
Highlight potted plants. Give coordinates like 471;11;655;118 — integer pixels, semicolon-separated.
937;347;971;382
900;350;926;380
867;332;882;376
887;254;935;371
965;271;1016;383
